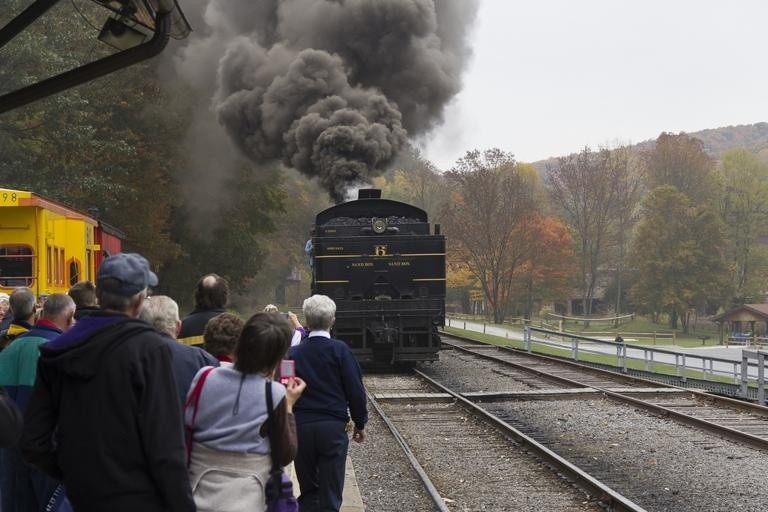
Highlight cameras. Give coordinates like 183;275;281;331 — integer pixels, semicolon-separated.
280;311;290;319
279;360;295;384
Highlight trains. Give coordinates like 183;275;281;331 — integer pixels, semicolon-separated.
309;187;447;371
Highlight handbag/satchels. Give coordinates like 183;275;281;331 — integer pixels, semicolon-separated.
266;469;299;512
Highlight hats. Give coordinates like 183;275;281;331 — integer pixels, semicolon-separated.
98;252;158;286
